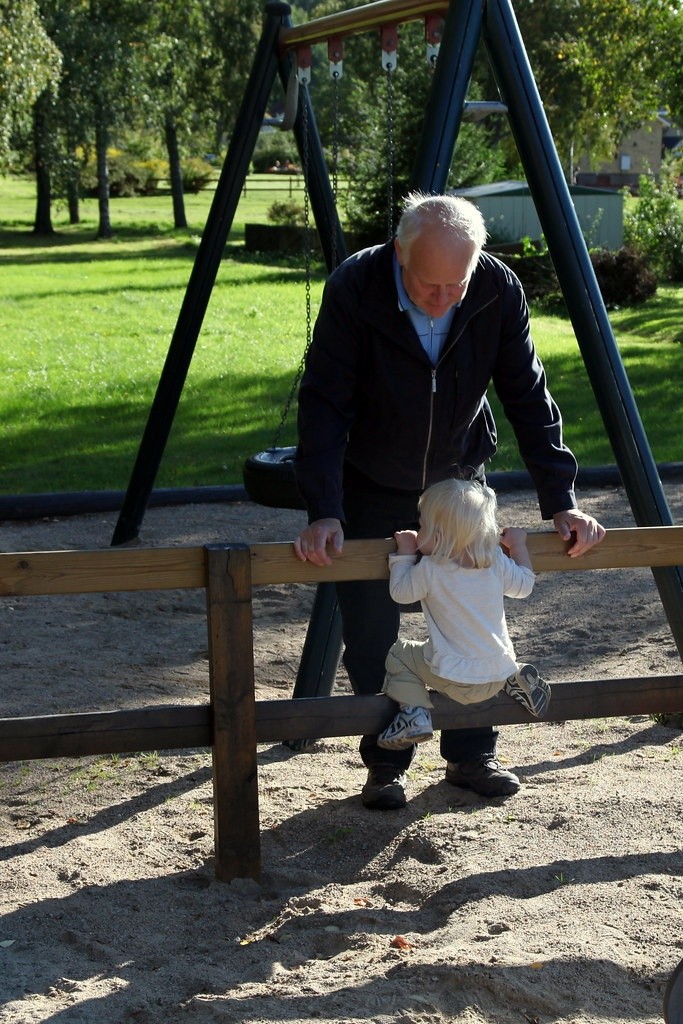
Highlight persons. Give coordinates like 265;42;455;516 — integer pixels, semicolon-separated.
376;479;552;752
293;195;606;810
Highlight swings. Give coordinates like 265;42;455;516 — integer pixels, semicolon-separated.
243;68;435;511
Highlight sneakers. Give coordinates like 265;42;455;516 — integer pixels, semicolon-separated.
445;731;521;797
378;706;434;751
361;764;408;809
504;660;552;718
